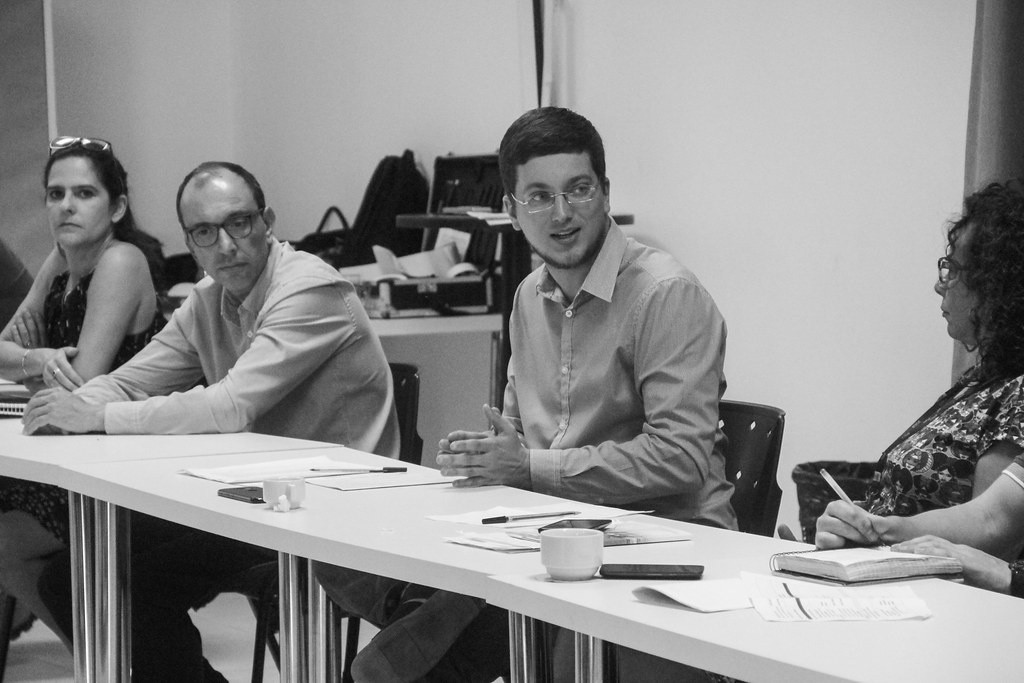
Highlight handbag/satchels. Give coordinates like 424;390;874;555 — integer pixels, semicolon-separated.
295;206;376;266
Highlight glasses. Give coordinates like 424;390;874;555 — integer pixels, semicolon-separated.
49;136;113;161
185;207;265;248
510;176;601;213
937;256;990;283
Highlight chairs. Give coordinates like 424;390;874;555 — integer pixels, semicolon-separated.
229;362;422;683
718;401;787;539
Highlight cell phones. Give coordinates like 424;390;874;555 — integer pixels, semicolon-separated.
538;519;613;531
218;486;264;503
600;564;704;579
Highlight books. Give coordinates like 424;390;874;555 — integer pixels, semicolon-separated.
771;544;962;588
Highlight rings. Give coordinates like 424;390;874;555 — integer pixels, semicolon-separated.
21;349;30;378
53;368;60;376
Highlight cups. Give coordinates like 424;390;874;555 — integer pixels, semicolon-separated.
541;526;605;583
262;477;306;511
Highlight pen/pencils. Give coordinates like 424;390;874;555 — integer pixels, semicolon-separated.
481;511;582;524
311;466;406;473
819;466;884;546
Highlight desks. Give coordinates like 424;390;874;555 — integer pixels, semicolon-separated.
0;417;1024;682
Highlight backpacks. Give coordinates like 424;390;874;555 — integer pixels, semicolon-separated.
352;150;429;256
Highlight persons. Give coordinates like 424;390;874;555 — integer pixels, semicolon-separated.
311;107;735;683
0;135;402;683
777;177;1024;601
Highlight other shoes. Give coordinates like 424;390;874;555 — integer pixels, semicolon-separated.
351;584;486;683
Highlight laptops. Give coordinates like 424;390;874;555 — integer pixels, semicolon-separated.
0;390;36;416
